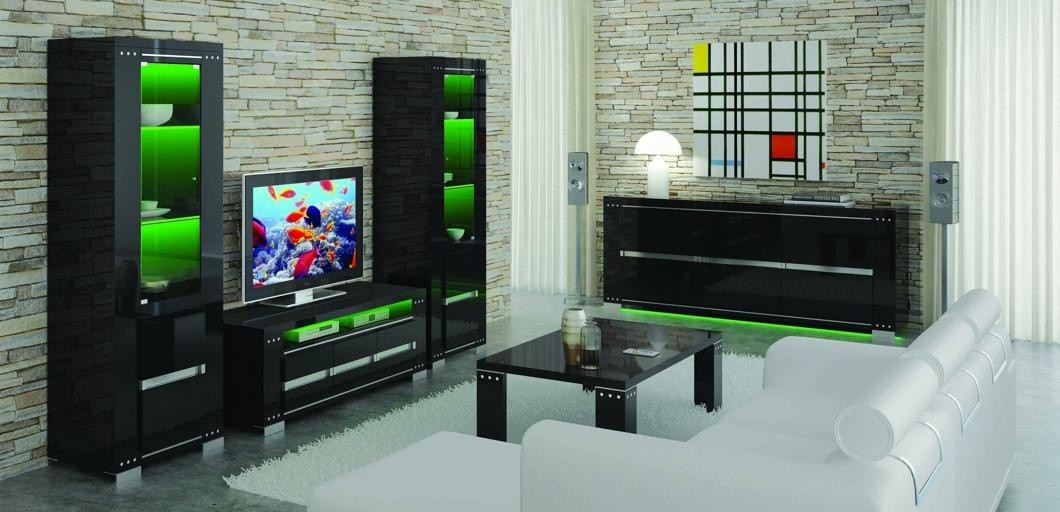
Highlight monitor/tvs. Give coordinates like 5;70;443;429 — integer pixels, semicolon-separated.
241;165;363;309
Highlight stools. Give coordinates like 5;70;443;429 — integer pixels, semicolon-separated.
309;427;522;512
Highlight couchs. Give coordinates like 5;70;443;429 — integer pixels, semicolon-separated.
518;288;1020;512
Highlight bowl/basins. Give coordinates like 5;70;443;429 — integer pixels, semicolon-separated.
141;200;158;210
446;227;465;241
445;111;459;119
140;103;174;127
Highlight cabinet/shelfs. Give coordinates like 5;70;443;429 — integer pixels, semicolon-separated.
601;194;909;345
45;35;225;487
370;54;487;369
223;280;428;439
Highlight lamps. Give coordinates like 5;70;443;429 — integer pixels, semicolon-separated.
632;130;683;200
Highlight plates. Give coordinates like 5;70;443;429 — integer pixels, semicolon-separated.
141;208;171;219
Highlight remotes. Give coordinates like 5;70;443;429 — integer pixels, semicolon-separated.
622;348;660;358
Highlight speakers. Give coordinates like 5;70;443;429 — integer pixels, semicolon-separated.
568;152;589;206
928;161;960;224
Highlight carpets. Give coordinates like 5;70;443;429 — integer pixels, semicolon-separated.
222;350;767;506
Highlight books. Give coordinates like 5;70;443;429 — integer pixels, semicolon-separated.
783;198;856;206
791;190;851;202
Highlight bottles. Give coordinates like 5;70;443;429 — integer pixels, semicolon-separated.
577;321;602;371
